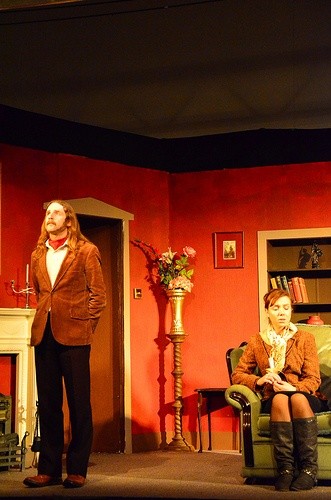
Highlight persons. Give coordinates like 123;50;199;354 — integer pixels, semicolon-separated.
22;199;107;488
311;241;322;268
232;290;321;492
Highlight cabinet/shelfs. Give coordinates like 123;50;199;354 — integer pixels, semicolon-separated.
257;227;331;331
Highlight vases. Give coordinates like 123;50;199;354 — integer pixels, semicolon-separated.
167;289;185;334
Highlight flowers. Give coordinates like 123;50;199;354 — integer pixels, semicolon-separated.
131;237;195;293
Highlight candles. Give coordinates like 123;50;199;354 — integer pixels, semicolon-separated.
26;263;29;281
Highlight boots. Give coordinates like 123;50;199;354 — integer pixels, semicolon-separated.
269;421;294;492
291;416;317;491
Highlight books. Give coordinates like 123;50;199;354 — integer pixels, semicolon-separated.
271;275;289;294
287;277;309;303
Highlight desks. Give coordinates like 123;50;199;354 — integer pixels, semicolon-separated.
194;388;242;454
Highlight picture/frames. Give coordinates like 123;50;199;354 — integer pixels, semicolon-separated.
213;231;245;269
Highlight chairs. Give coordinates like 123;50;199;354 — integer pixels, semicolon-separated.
226;323;331;483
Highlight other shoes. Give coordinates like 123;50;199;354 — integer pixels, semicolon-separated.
63;473;86;488
23;474;63;487
